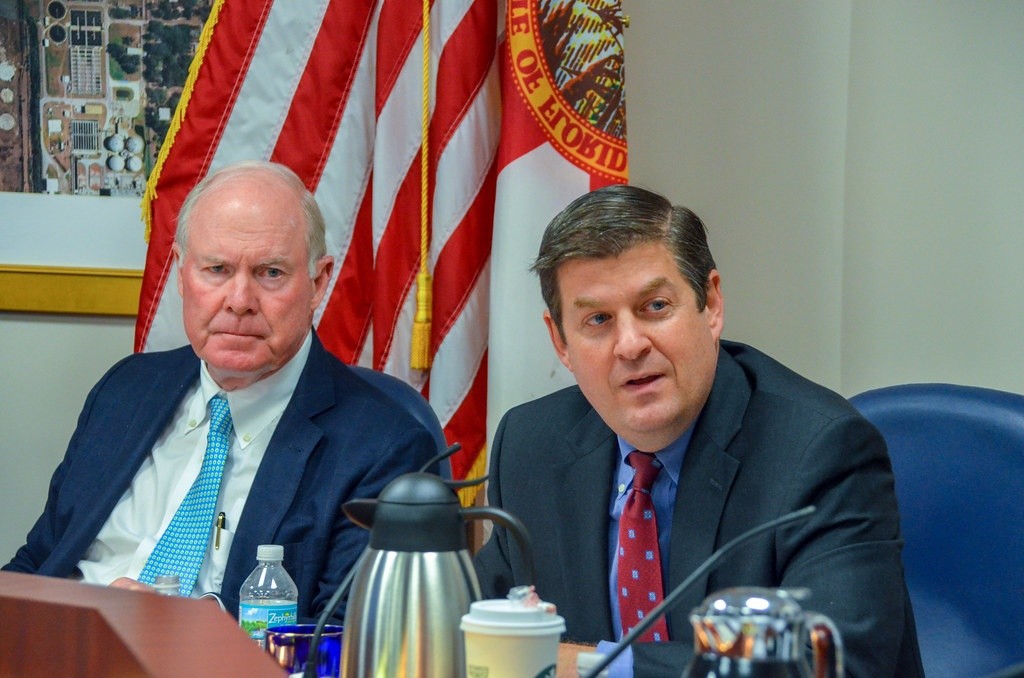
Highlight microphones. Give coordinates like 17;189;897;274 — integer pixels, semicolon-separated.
581;506;818;678
303;442;463;678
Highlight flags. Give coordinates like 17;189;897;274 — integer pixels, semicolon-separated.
136;1;627;519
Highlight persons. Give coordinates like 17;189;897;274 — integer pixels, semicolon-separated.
0;161;443;626
472;183;926;677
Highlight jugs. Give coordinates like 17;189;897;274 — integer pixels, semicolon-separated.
680;586;845;678
339;472;535;678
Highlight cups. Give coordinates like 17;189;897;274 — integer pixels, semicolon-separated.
459;585;566;678
265;624;343;678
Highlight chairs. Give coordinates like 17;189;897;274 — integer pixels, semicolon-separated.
851;382;1024;678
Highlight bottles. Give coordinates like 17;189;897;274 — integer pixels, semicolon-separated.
238;544;298;650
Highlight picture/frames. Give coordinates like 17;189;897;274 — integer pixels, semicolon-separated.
0;2;219;318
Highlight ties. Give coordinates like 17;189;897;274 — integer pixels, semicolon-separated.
616;450;670;642
136;395;234;596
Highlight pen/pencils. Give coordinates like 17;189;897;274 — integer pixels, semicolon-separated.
215;510;226;550
